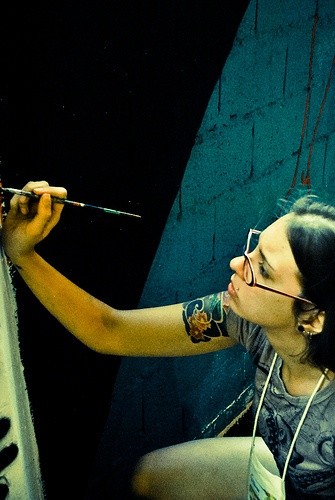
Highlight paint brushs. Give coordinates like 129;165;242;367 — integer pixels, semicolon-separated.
1;187;142;219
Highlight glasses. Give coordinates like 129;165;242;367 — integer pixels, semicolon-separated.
242;228;317;309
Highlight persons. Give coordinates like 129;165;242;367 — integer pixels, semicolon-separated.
3;179;335;499
